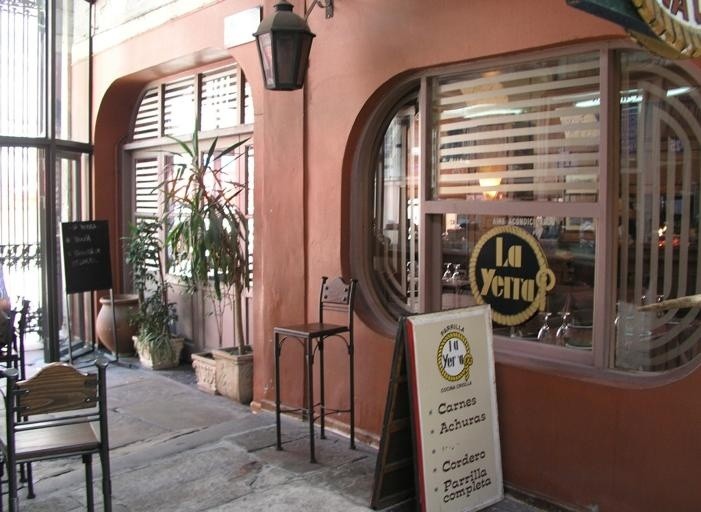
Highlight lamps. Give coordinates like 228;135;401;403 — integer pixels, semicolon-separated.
251;0;337;91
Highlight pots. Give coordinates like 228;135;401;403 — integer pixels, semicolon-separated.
566;321;593;347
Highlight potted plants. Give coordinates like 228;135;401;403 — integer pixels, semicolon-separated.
117;131;258;404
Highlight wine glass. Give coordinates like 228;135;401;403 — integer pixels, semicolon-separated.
441;261;452;281
557;311;569;338
538;311;553;341
451;263;460;281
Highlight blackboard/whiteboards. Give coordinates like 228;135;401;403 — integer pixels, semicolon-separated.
61;219;113;294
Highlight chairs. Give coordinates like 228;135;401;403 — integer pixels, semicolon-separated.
0;361;110;511
270;275;360;465
0;298;30;426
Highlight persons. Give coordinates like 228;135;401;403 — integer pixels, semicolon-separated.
1;265;13;349
